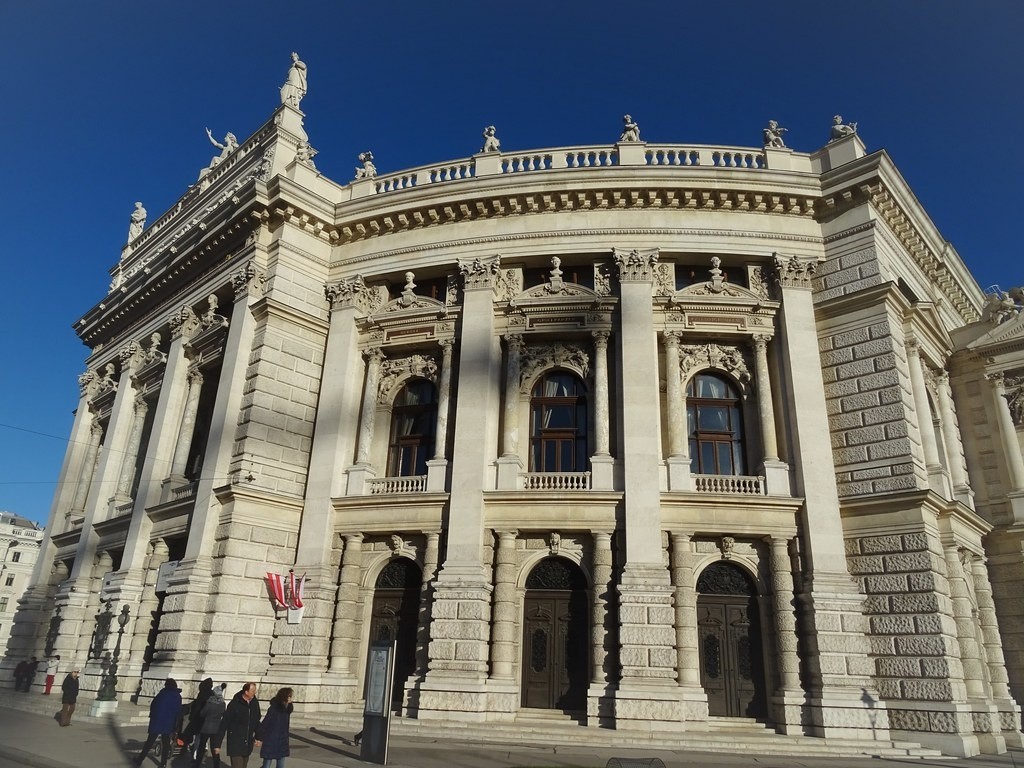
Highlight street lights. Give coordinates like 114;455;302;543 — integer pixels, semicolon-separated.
96;603;133;699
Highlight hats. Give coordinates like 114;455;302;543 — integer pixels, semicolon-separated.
213;683;227;698
72;667;79;672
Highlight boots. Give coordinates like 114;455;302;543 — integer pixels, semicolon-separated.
213;754;220;768
191;750;207;768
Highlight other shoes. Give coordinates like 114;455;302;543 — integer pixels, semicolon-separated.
42;692;50;695
60;722;71;727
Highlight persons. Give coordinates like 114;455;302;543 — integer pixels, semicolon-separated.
481;126;501;152
132;677;295;768
151;332;161;345
708;256;723;275
58;667;80;726
280;51;307;109
403;271;417;292
828;114;854;142
549;533;561;545
205;126;239;169
993;291;1018;327
128;202;146;243
721;536;734;551
208;294;217;307
13;655;60;694
355;152;374;178
549;256;563;277
390;533;404;550
619;115;640;141
763;120;788;148
105;363;115;373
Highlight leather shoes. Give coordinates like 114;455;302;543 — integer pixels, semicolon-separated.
157;764;166;768
132;756;141;766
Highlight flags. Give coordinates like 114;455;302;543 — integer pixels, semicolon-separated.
265;572;306;609
